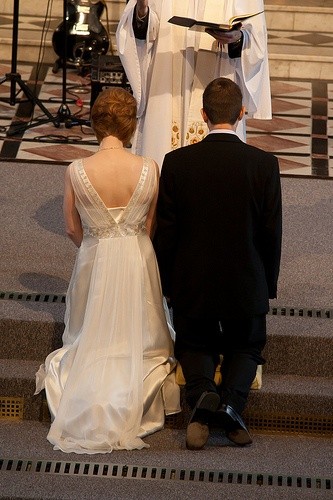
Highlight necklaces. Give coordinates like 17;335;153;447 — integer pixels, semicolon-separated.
98;146;124;150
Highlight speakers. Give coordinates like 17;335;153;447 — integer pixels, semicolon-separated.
90;54;133;112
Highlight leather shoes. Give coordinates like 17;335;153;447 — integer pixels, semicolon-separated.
186;391;220;451
218;404;253;446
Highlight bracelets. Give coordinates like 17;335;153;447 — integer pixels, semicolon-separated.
136;5;149;22
233;39;240;45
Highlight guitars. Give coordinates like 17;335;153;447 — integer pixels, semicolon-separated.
51;0;112;62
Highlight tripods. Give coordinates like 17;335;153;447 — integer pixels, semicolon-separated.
1;0;90;135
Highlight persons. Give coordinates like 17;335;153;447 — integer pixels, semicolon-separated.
152;77;283;451
116;0;272;175
33;87;186;455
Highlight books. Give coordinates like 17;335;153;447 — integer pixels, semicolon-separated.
168;10;264;32
